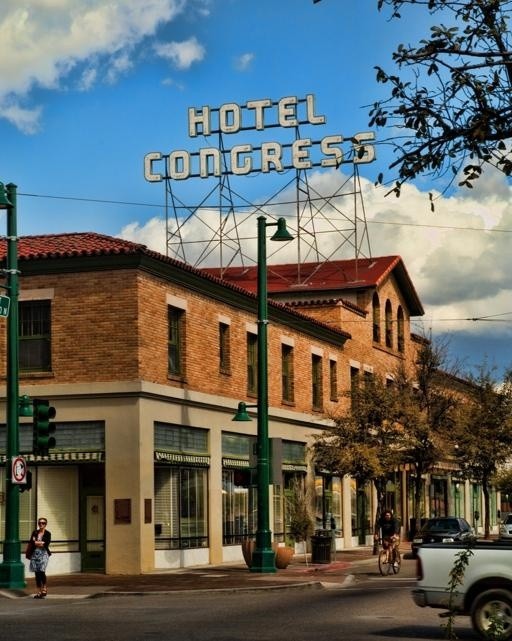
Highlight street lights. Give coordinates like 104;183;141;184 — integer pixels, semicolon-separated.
1;181;27;590
248;216;295;574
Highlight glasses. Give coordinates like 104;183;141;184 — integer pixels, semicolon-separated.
38;522;46;525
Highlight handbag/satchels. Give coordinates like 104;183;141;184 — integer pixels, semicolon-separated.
26;542;34;559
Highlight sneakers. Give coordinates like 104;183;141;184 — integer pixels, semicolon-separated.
394;562;398;567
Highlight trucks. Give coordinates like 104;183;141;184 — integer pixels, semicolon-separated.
412;538;512;640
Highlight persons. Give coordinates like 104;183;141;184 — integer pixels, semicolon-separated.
27;519;52;599
373;510;402;569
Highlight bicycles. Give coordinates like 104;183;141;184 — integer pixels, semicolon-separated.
374;537;401;576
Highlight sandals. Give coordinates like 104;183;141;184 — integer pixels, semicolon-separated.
34;588;47;598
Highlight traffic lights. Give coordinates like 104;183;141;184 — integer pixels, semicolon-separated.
31;398;57;456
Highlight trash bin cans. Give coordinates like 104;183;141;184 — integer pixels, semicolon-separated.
409;518;429;542
310;535;333;564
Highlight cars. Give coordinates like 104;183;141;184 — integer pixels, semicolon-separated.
410;516;473;559
497;514;512;542
